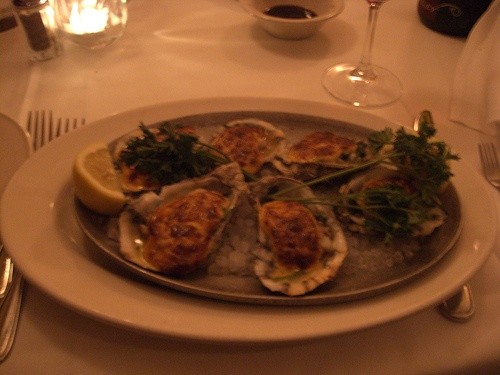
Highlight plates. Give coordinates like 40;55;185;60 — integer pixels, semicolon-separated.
0;94;500;344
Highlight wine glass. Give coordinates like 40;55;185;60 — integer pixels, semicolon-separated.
320;0;405;107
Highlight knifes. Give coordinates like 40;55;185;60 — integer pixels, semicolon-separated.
415;110;475;323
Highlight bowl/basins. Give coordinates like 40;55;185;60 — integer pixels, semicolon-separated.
239;0;346;39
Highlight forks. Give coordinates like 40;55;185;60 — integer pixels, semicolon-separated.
0;110;87;360
478;142;500;190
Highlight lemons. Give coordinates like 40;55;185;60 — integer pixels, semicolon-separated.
73;141;126;214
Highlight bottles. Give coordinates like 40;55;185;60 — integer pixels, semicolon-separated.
12;0;65;62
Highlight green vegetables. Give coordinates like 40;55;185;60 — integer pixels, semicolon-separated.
117;114;461;249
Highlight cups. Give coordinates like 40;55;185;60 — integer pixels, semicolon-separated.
53;0;130;52
